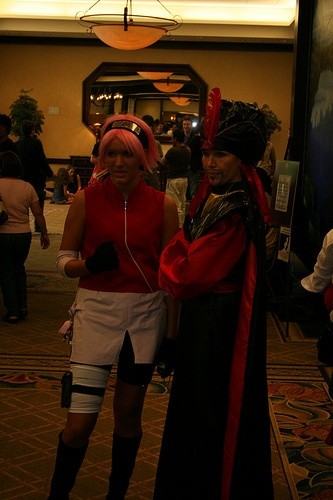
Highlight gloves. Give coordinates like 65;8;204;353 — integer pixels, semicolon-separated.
86;241;119;274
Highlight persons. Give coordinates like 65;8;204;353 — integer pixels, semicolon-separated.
0;115;50;322
151;100;283;500
300;227;333;366
46;114;180;500
140;111;200;189
254;142;278;270
51;165;82;205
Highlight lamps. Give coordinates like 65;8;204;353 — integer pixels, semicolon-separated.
139;72;190;107
75;0;184;51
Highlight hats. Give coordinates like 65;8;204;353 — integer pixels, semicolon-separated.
201;87;281;167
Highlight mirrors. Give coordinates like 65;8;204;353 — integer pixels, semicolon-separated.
80;61;209;140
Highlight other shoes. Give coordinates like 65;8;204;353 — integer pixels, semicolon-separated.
7;310;27;323
